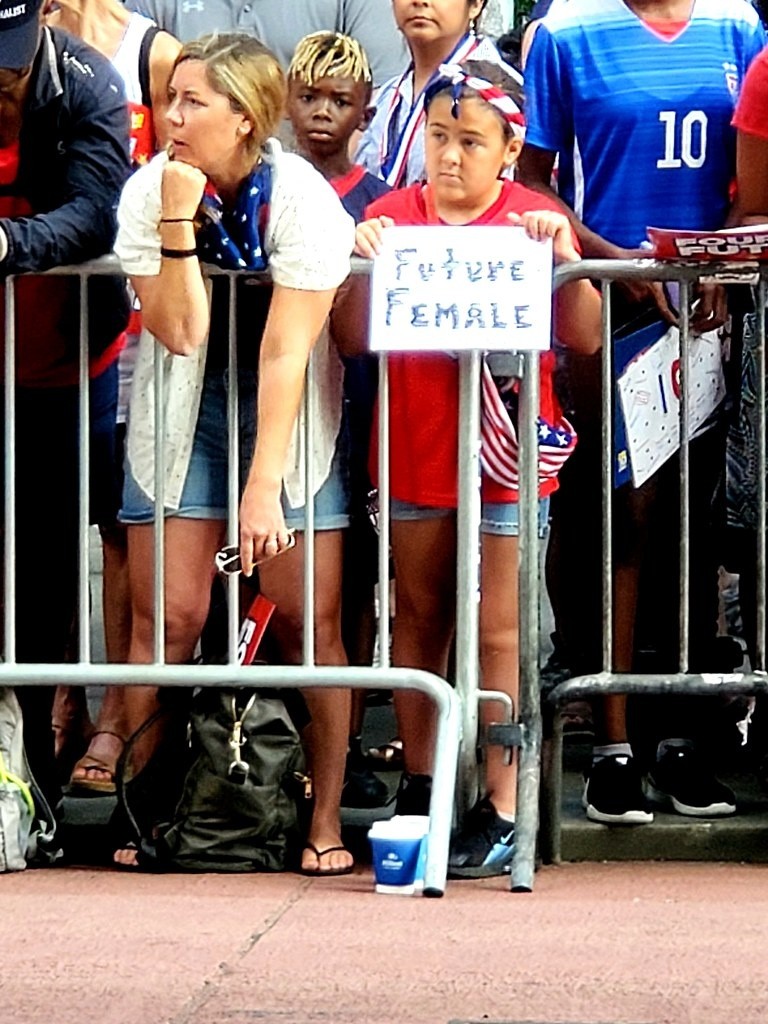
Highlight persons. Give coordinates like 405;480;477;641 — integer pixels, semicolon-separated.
111;35;354;878
0;0;768;879
332;57;607;878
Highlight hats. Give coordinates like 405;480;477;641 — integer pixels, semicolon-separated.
0;0;43;68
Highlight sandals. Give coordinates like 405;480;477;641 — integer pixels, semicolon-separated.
70;731;133;792
300;839;354;875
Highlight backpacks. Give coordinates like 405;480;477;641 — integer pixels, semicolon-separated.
0;686;64;871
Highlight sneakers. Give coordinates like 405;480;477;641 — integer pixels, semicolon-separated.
648;744;737;815
384;771;431;815
583;752;654;823
448;799;516;876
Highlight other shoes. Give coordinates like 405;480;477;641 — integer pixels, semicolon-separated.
562;701;595;733
342;758;389;802
364;735;406;768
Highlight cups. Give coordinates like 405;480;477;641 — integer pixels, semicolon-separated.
366;815;430;896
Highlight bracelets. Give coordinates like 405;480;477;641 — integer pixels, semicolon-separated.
161;219;194;222
161;246;198;259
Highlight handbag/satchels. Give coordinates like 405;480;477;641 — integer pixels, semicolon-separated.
115;655;311;874
